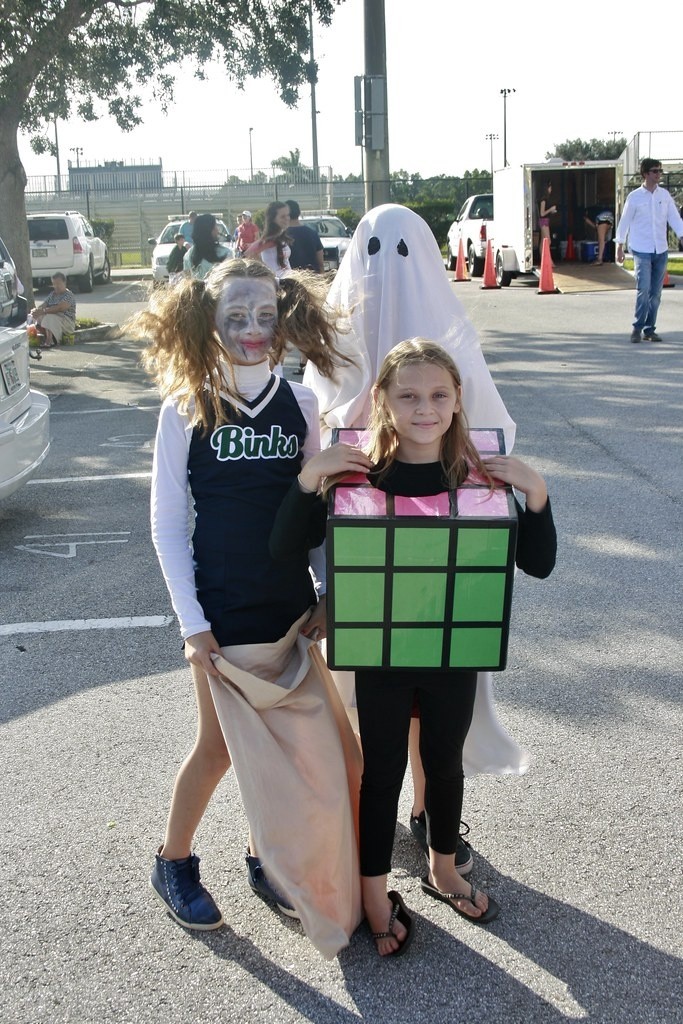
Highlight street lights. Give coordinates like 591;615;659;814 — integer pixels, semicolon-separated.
607;131;623;158
498;87;516;165
67;146;84;170
249;127;255;184
484;134;500;175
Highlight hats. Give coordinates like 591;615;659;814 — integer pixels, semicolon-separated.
242;211;252;217
188;211;197;219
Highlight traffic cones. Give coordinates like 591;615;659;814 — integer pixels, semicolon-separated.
451;238;472;282
478;239;502;289
533;237;560;295
562;231;578;263
663;267;676;289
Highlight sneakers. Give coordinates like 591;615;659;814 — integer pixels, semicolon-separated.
243;846;300;919
149;843;223;931
410;810;472;875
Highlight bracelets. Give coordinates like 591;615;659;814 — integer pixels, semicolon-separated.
43;308;46;314
297;474;318;492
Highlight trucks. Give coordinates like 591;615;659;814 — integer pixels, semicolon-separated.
492;158;639;294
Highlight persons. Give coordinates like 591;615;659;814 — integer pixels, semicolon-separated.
183;214;234;278
302;203;527;876
31;271;76;348
613;159;683;343
282;200;325;274
125;254;327;928
178;211;197;250
234;210;261;258
583;206;614;266
268;340;558;956
539;180;559;267
243;200;291;276
166;234;188;273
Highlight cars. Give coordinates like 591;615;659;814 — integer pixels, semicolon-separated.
0;235;53;506
297;205;354;272
147;213;239;289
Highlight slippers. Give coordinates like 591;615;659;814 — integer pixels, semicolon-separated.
421;875;498;924
372;890;415;956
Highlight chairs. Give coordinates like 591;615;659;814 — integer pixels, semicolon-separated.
475;208;489;219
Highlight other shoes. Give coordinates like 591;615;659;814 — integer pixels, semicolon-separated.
293;363;305;374
631;328;641;343
40;342;54;348
591;261;602;266
643;333;661;341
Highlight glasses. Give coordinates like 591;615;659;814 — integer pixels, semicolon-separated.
649;169;663;173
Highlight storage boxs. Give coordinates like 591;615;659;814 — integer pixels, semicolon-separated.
581;240;609;263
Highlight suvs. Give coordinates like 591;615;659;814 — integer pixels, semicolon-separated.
27;210;113;294
445;193;496;277
